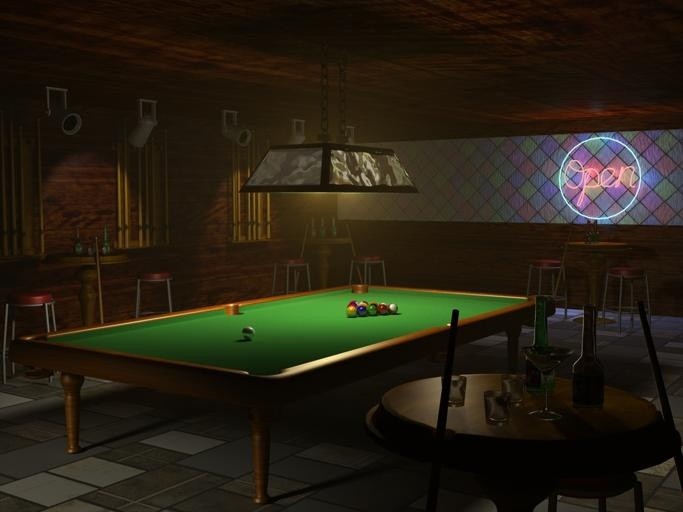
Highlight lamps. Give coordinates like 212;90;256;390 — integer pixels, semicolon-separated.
222;108;252;147
44;86;82;135
238;0;421;192
128;99;159;148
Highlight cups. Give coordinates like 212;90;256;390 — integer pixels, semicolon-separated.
444;374;466;407
502;373;528;409
483;390;511;426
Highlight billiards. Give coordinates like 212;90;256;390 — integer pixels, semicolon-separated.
242;326;255;340
347;298;397;317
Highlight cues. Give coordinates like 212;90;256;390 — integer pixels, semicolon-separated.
638;299;682;492
300;223;310;259
345;224;362;284
427;309;459;512
554;228;572;295
95;235;104;324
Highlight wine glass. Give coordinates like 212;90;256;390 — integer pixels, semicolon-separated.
521;344;574;420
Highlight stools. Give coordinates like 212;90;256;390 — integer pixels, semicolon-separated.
3;291;59;384
272;260;312;295
527;259;567;319
601;266;651;336
349;256;386;286
136;272;175;319
546;472;644;511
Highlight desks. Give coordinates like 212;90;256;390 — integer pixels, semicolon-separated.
363;373;682;512
296;236;351;289
556;241;634;326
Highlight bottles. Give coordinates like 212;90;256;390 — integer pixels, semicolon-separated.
86;235;95;256
572;303;605;407
583;219;599;245
309;217;316;239
329;216;336;239
319;217;325;239
71;226;82;256
101;222;111;255
523;295;553;392
